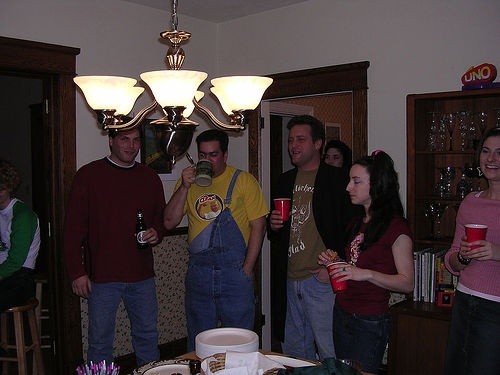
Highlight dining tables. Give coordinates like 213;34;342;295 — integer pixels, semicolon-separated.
125;349;378;375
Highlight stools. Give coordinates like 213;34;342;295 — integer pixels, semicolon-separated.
0;297;46;375
33;279;51;348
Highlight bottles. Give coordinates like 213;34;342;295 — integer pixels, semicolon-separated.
136;209;149;248
457;171;471;198
190;360;201;375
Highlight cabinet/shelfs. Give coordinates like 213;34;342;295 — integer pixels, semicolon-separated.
391;87;500;375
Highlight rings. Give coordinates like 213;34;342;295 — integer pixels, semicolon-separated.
343;267;346;272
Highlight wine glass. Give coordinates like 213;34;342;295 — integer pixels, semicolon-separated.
443;112;459;151
477;111;487;138
457;112;473;151
426;112;442;151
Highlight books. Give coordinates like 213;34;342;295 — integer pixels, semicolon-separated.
411;247;459;302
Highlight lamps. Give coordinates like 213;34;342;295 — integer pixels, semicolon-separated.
73;0;273;168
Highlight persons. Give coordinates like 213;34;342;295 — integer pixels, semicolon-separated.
317;149;413;375
0;157;41;356
163;129;270;352
267;115;365;362
323;140;352;169
61;116;167;367
444;129;500;375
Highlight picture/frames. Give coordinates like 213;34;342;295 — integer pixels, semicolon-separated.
132;112;177;181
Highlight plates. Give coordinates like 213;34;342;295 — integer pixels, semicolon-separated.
196;328;259;359
129;359;205;375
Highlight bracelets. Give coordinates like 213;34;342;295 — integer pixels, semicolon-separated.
457;251;471;265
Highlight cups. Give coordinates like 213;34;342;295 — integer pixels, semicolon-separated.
274;199;290;221
464;224;488;250
327;262;347;292
191;160;213;186
